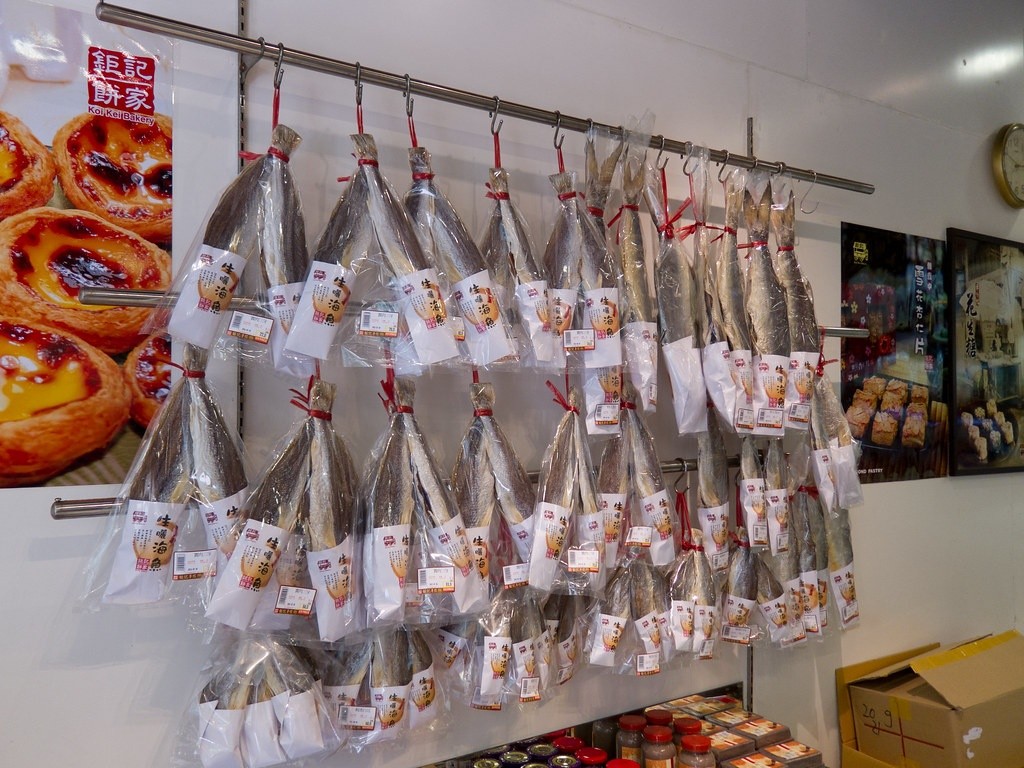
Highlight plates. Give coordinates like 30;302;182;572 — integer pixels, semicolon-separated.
955;399;1019;466
841;371;935;459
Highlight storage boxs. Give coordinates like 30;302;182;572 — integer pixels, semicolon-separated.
833;628;1024;768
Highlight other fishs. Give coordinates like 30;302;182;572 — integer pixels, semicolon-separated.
116;124;860;708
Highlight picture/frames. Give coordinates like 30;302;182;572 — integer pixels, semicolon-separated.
945;226;1024;478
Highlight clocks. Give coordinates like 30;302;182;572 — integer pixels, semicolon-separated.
992;122;1024;209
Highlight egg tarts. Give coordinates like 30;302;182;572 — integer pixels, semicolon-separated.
0;107;173;488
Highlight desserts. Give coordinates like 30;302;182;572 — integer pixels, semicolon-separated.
844;375;1014;460
